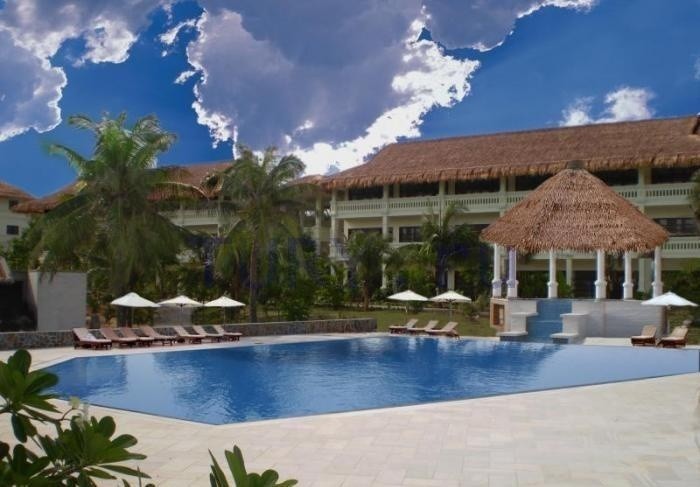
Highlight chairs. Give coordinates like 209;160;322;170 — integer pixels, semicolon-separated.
68;320;244;350
630;323;657;347
388;315;462;338
658;324;689;349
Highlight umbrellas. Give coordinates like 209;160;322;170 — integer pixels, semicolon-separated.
110;292;160;328
156;294;204;324
204;295;246;331
429;290;472;323
639;289;699;334
386;289;428;325
